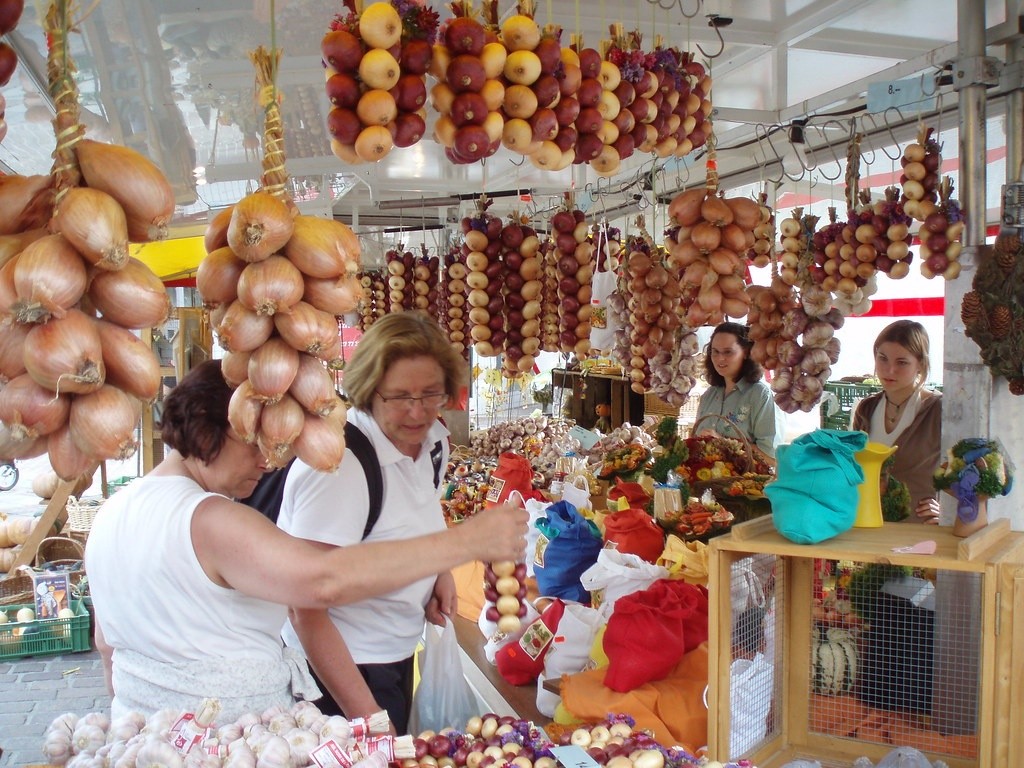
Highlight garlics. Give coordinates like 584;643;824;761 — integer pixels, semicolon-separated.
606;276;878;413
42;701;391;768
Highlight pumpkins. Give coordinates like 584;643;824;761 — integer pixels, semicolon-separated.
32;468;92;499
0;606;75;640
809;628;859;695
0;512;58;572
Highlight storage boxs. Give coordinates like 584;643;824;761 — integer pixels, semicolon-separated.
106;476;134;496
0;578;94;662
821;381;882;431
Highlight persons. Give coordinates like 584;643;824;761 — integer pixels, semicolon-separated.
274;312;460;735
694;323;776;457
853;320;943;526
83;358;529;730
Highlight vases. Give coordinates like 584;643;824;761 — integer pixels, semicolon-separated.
943;489;990;538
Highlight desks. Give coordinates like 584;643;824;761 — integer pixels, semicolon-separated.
418;612;551;725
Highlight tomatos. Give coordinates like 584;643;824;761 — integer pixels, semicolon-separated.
812;598;863;628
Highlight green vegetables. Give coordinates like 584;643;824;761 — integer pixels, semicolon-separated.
848;562;912;622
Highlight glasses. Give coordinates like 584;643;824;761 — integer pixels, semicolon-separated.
373;388;450;409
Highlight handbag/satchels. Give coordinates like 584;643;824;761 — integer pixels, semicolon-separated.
406;611;482;737
477;451;779;761
763;428;867;545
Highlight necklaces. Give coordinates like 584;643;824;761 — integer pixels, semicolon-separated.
887;395;912;423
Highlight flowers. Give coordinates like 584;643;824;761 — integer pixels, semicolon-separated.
596;417;775;500
820;560;923;624
933;438;1007;497
879;458;910;525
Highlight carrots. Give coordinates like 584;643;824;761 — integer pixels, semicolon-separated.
677;512;716;531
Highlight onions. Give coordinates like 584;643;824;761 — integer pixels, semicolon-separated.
1;0;709;472
484;554;527;633
663;138;964;327
383;713;729;768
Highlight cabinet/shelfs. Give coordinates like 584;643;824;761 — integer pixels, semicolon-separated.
553;367;645;435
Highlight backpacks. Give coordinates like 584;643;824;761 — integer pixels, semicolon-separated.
233;420;444;543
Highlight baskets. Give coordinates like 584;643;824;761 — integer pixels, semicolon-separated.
0;534;95;663
677;413;776;499
65;493;106;532
